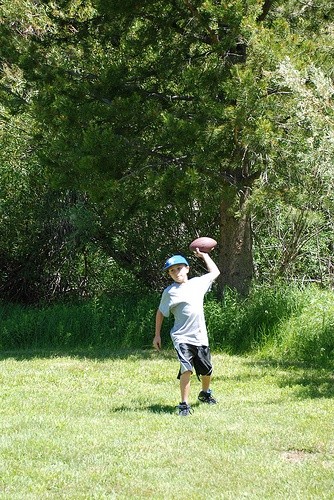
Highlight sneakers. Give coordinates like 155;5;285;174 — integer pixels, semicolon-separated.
198;389;218;404
178;402;192;418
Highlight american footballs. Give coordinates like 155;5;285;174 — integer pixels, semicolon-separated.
189;237;217;253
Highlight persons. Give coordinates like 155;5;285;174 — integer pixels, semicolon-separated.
153;247;221;416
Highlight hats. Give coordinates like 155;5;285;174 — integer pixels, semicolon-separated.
164;255;189;270
